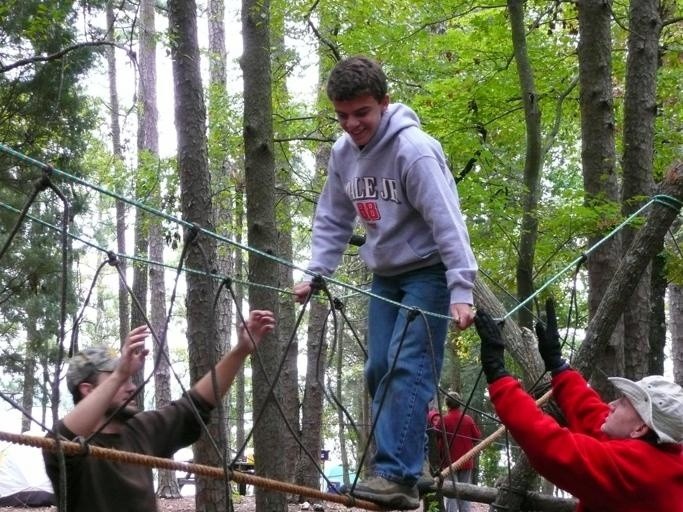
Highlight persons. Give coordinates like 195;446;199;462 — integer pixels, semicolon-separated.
289;54;480;504
43;307;278;511
432;390;480;512
472;297;683;511
423;406;443;469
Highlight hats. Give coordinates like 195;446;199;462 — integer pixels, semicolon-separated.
66;346;121;394
605;372;683;448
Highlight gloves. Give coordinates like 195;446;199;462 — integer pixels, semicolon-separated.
472;308;511;385
535;296;567;372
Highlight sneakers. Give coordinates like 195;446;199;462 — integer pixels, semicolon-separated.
312;503;324;511
300;500;311;510
345;470;422;511
